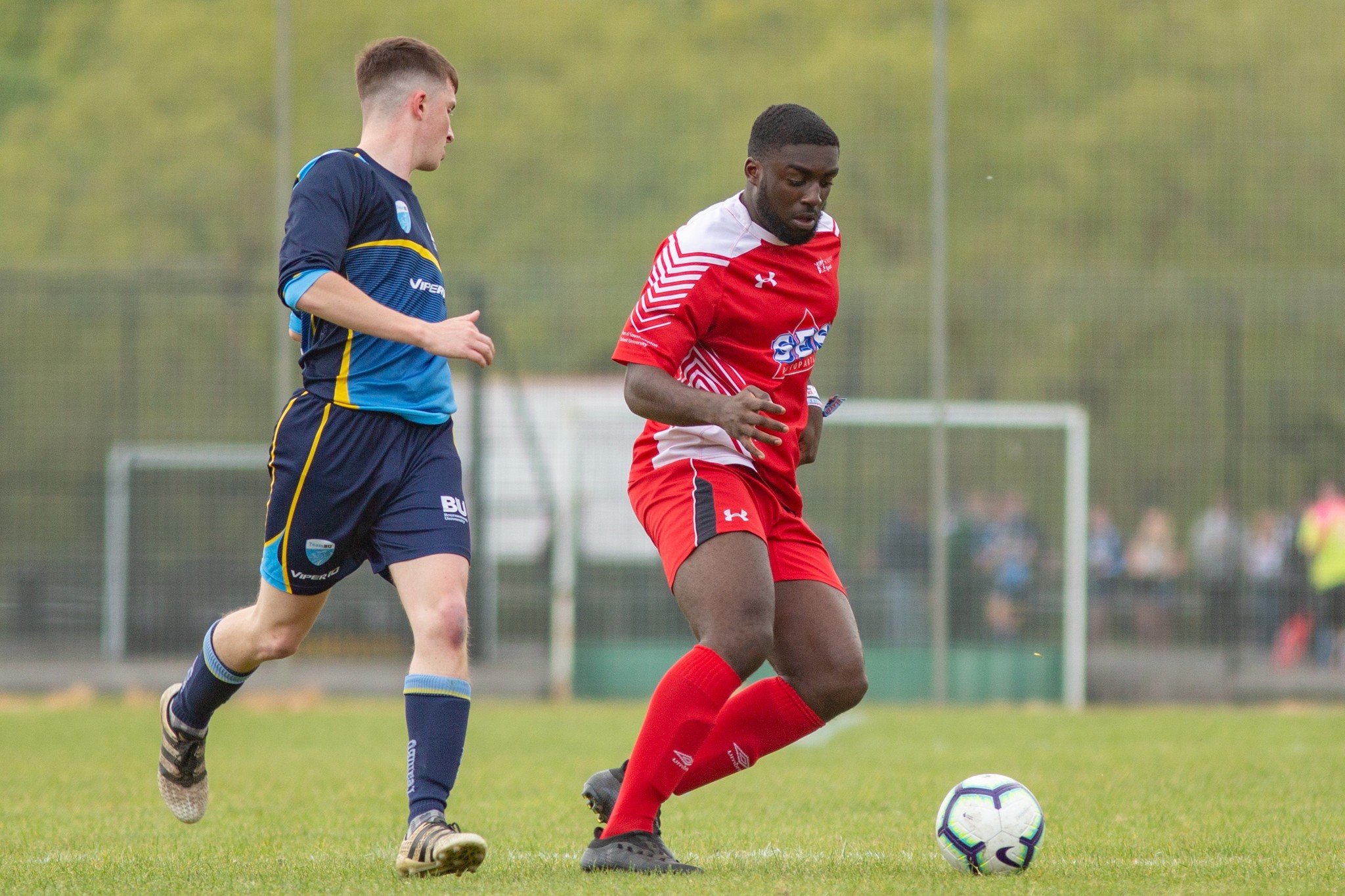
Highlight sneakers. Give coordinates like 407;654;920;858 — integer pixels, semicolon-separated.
393;820;488;878
582;760;663;839
158;683;210;824
579;826;705;878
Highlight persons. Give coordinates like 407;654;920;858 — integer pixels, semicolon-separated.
160;37;489;878
883;472;1344;689
580;105;870;874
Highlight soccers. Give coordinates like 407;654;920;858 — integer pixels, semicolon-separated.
935;774;1046;877
